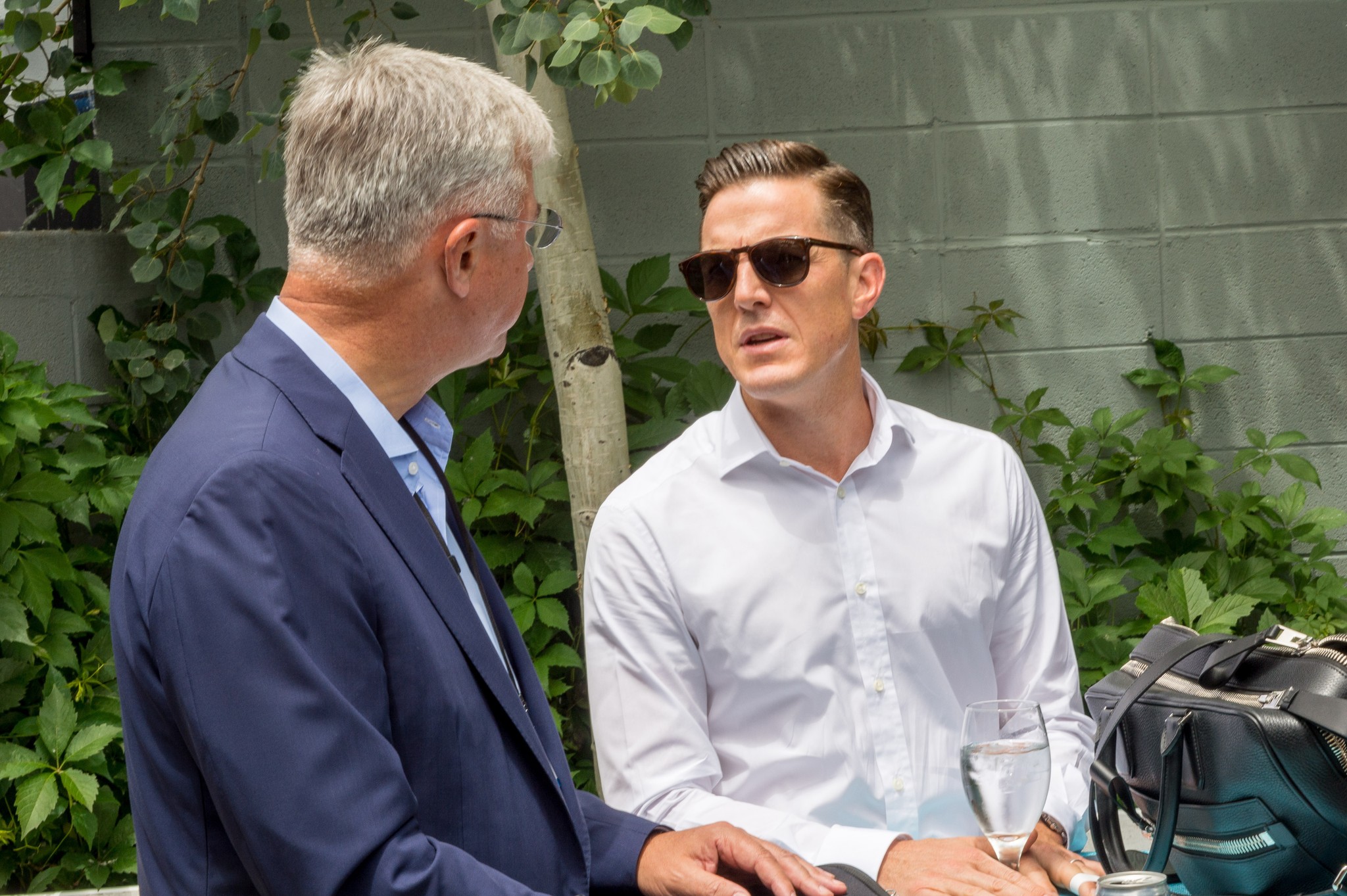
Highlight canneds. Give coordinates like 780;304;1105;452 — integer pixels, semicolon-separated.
1093;870;1172;896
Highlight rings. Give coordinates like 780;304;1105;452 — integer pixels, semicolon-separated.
1070;859;1085;866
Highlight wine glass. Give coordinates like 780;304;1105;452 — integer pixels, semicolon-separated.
960;700;1051;872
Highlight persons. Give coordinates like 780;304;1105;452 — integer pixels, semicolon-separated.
581;138;1098;896
111;34;890;896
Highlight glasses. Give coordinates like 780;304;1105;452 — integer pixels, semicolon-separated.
470;201;564;250
678;234;865;302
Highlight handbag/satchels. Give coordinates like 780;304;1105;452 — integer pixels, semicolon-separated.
1083;618;1347;896
